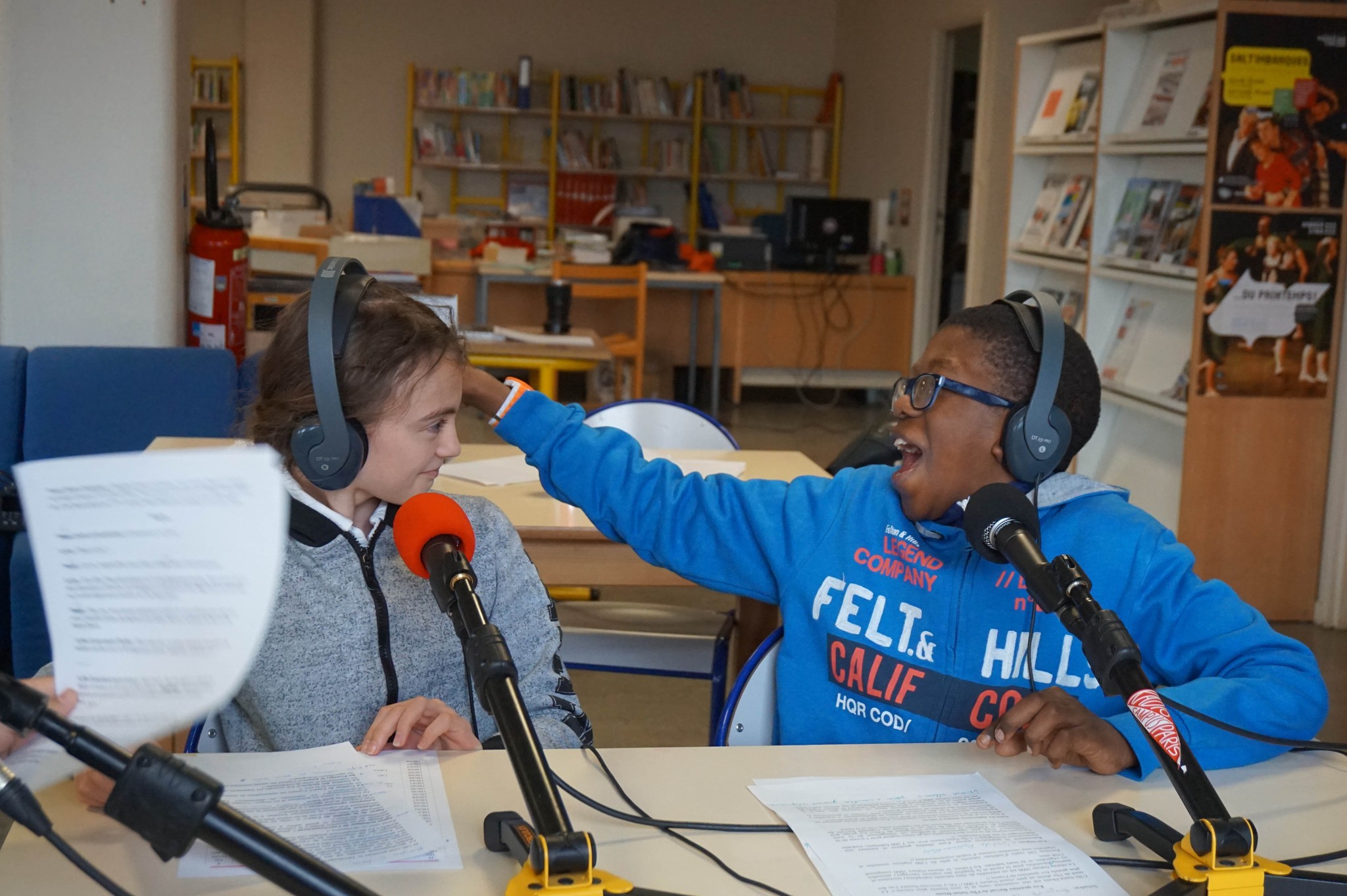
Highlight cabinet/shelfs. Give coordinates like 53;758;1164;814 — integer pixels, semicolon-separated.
999;0;1347;624
187;55;247;233
403;58;843;253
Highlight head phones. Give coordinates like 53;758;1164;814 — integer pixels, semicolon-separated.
987;289;1072;484
291;252;377;492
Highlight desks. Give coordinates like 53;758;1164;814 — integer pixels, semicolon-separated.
474;260;724;424
144;432;835;701
715;268;914;407
458;337;615;405
246;267;422;360
0;738;1347;896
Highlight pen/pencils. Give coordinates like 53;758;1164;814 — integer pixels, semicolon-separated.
549;587;601;600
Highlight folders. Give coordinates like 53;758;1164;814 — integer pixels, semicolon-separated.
518;54;531;109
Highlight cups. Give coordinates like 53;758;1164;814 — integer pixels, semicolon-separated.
544;277;571;335
871;253;885;275
885;251;899;277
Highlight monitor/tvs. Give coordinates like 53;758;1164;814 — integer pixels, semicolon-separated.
785;196;872;262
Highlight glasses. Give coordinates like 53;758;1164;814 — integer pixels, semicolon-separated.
890;372;1014;410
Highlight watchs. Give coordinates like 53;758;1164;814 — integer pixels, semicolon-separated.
484;374;536;429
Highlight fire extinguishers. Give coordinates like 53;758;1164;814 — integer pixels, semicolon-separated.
185;182;331;365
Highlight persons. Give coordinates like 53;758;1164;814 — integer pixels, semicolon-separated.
72;276;594;809
0;677;81;769
1201;82;1346;398
457;304;1329;784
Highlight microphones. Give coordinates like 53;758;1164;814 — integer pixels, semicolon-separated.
962;484;1083;637
394;492;493;714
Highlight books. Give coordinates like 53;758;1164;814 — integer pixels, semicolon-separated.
1010;42;1215;404
411;60;839;182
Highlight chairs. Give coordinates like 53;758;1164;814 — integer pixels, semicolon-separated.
0;342;275;713
543;262;650;416
716;623;787;749
550;399;742;749
